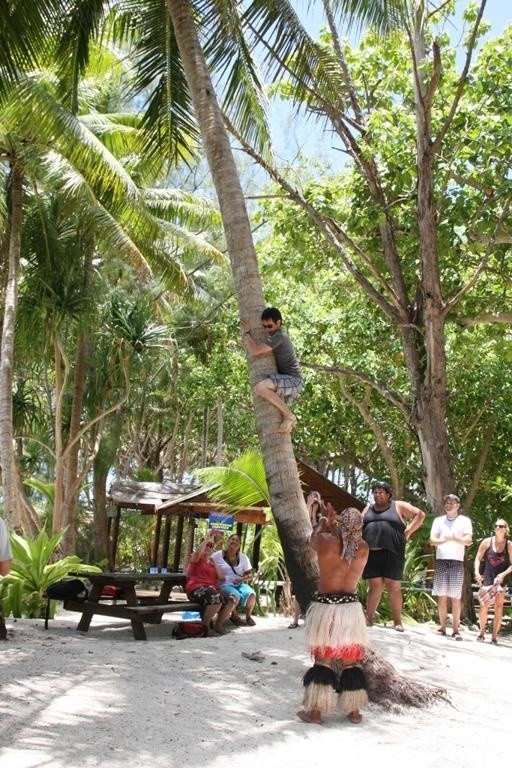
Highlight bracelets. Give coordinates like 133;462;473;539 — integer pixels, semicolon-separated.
242;331;250;334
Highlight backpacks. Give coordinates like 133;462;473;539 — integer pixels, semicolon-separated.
46;579;88;603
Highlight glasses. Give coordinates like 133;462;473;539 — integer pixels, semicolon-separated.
262;322;277;329
493;524;506;529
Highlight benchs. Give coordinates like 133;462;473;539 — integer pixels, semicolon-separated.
65;595;204;640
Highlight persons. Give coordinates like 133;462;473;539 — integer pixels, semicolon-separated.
0;515;13;577
240;307;304;433
427;494;474;640
294;501;370;725
209;534;257;635
185;529;237;635
474;518;512;648
287;490;329;629
361;483;426;632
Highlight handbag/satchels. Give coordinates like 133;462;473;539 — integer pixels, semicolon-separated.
172;621;209;640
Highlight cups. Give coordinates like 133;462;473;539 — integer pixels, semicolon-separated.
150;567;167;574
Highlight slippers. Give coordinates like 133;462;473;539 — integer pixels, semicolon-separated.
203;624;230;638
228;616;247;627
245;616;257;627
489;639;498;645
475;635;486;642
287;623;299;629
432;629;447;636
363;616;405;633
451;632;464;641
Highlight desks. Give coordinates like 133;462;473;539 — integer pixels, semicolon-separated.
69;572;204;639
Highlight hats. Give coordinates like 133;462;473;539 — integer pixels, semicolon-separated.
443;493;461;504
